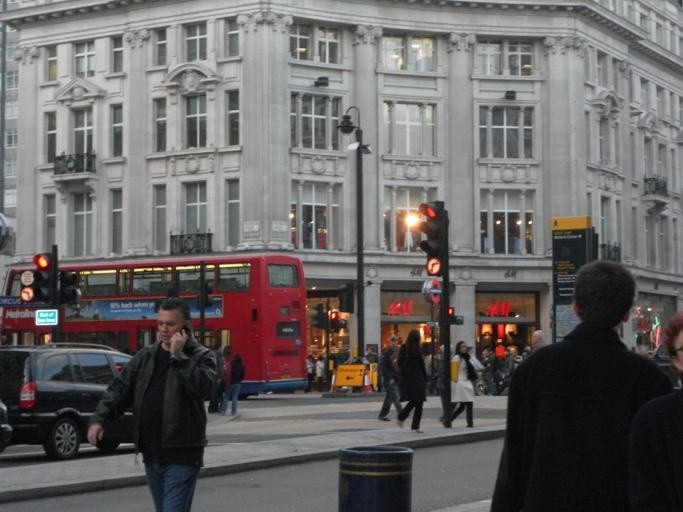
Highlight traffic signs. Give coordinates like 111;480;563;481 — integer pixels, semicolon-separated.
447;316;463;324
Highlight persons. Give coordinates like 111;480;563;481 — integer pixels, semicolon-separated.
219;354;245;416
489;260;672;512
629;312;683;512
87;297;219;512
304;330;546;434
208;344;225;414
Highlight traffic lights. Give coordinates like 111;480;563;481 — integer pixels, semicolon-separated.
418;200;446;256
426;255;443;276
312;303;328;331
58;269;81;305
29;251;53;302
328;310;339;332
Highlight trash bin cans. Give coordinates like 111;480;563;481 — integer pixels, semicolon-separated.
339;445;414;512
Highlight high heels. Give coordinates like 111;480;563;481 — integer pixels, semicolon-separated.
412;425;424;433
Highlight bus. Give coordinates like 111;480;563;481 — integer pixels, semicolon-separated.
0;250;311;398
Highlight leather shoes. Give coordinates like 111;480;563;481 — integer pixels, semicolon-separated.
378;415;392;421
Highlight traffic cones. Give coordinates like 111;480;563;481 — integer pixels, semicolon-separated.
357;369;374;394
328;368;336;393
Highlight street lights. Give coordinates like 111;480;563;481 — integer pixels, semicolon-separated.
338;103;372;364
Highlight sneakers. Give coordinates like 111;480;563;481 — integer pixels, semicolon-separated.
220;410;238;416
396;414;403;428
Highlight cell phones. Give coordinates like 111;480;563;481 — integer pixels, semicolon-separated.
180;326;190;337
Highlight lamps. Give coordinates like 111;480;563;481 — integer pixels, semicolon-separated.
315;77;329;86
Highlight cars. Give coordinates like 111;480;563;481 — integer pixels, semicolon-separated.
643;339;678;383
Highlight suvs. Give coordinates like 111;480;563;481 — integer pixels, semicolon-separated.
0;342;139;460
